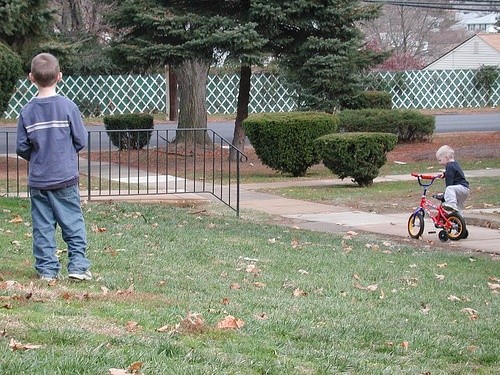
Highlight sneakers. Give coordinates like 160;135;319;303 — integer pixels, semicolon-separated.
40;273;64;282
68;269;93;283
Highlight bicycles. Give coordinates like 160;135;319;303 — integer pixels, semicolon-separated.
408;173;468;242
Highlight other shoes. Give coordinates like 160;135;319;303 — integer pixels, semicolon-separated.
442;206;454;211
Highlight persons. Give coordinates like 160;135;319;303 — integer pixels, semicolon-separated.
435;145;470;218
16;53;93;280
